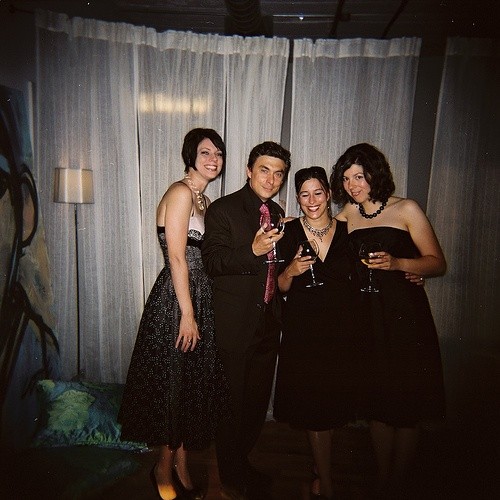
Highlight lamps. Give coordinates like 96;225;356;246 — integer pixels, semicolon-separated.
52;168;95;379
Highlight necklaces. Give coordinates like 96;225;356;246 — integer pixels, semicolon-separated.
359;198;388;219
184;172;205;210
303;215;333;242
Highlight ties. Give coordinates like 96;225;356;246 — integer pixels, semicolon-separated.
259;203;276;304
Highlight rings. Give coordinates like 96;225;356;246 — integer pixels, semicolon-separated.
420;278;423;281
187;339;191;342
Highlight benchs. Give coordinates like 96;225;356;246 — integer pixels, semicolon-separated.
0;427;158;500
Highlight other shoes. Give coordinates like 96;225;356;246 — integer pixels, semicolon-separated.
220;465;273;500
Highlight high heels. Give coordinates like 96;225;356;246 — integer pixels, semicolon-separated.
309;463;340;500
170;466;203;500
150;463;181;500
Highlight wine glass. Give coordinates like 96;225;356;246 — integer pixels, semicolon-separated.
298;239;323;287
359;242;384;292
260;213;285;264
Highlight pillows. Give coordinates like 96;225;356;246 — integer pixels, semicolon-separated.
32;376;150;453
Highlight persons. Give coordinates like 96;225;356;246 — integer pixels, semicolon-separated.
276;166;426;500
201;142;296;491
280;142;447;500
117;128;239;500
